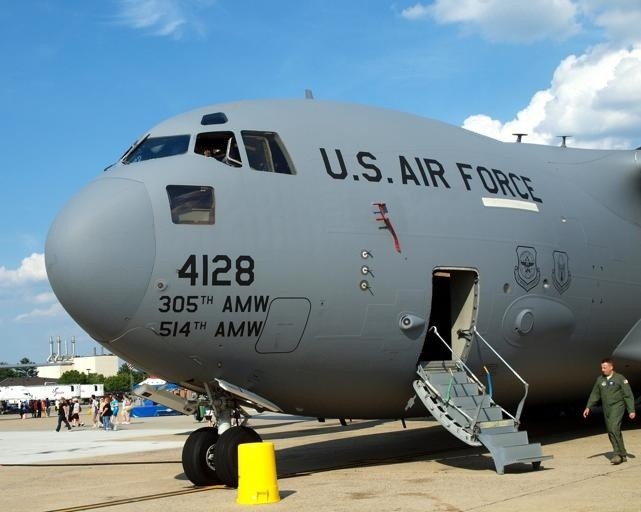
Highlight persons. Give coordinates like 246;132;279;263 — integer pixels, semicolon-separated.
203;147;211;159
1;389;144;432
583;356;637;463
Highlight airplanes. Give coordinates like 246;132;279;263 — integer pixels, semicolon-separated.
41;90;640;486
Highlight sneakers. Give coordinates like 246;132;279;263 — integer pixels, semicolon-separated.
612;455;627;465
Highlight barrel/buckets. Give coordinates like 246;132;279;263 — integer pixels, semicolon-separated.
235;442;282;506
